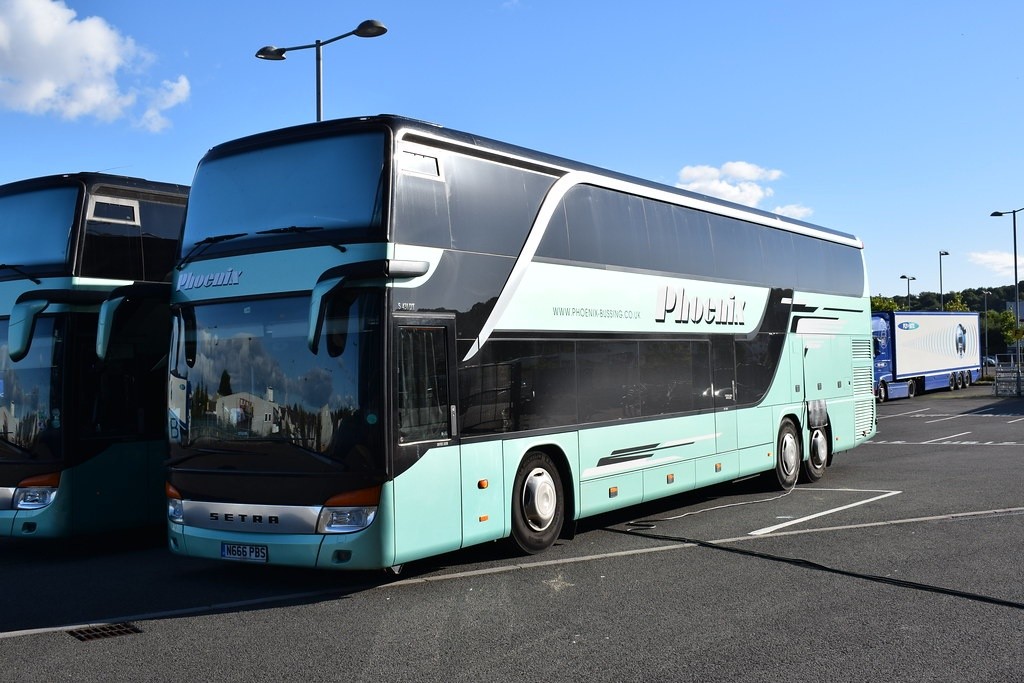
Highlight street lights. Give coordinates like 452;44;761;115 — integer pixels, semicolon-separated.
900;275;916;311
990;209;1024;397
939;251;949;311
982;290;992;375
256;19;388;121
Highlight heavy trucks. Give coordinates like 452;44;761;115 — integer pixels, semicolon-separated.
870;309;983;402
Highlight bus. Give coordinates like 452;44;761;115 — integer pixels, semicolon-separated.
96;113;878;579
0;171;193;549
